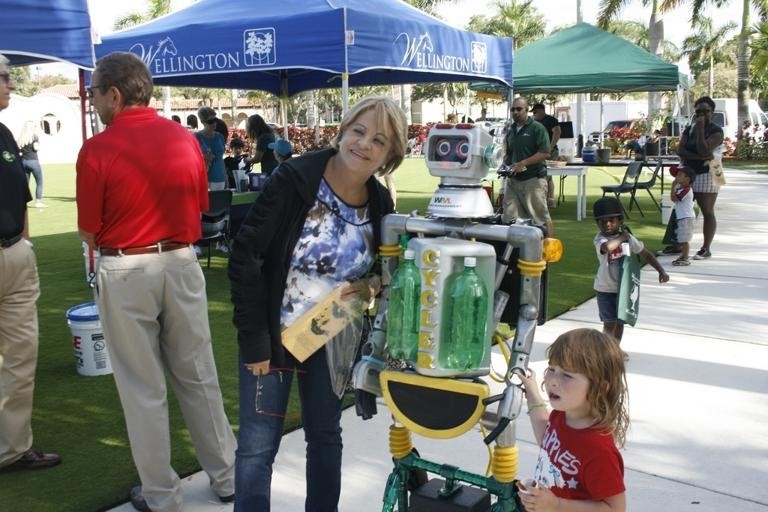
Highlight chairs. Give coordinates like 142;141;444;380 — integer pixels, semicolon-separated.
200;190;231;268
635;160;663;213
601;161;645;219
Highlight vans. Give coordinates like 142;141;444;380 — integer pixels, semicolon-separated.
657;115;694;154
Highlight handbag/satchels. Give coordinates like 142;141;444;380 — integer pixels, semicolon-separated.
617;224;642;328
662;208;679;245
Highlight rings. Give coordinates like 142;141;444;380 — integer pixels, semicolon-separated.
246;366;250;372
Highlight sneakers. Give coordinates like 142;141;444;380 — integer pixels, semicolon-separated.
215;241;232;253
34;198;49;208
547;197;557;208
194;244;204;256
656;247;682;256
672;256;691;266
693;247;712;260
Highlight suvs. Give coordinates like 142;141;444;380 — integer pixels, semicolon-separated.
588;119;663;149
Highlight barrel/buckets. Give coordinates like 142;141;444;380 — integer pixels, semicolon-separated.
661;193;675;225
596;148;610;164
66;301;113;377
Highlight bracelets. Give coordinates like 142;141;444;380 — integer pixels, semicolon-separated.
25;239;33;247
526;402;548;415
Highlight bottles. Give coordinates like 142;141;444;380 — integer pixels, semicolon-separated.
388;251;417;363
446;257;488;371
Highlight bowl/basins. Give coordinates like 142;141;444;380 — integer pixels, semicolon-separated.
548;161;568;169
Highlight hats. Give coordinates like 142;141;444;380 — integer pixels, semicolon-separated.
267;139;292;157
528;104;545;112
592;195;623;220
670;165;696;185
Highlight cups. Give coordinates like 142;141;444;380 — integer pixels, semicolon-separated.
233;169;248;193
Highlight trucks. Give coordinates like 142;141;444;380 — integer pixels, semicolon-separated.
711;98;768;155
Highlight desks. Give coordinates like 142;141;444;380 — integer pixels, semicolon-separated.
547;166;587;221
556;157;680;205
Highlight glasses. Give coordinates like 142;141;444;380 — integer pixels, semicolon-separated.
510;106;527;113
203;118;218;125
695;109;712;114
254;367;308;419
87;85;110;98
0;72;11;81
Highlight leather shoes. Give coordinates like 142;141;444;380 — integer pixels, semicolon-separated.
130;485;155;512
219;492;237;504
0;450;63;473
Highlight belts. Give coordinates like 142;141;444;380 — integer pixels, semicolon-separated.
99;239;195;259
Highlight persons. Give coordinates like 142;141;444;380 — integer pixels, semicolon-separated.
511;328;630;512
17;120;48;208
77;51;239;512
0;53;62;473
224;96;405;511
592;195;670;339
669;164;697;265
